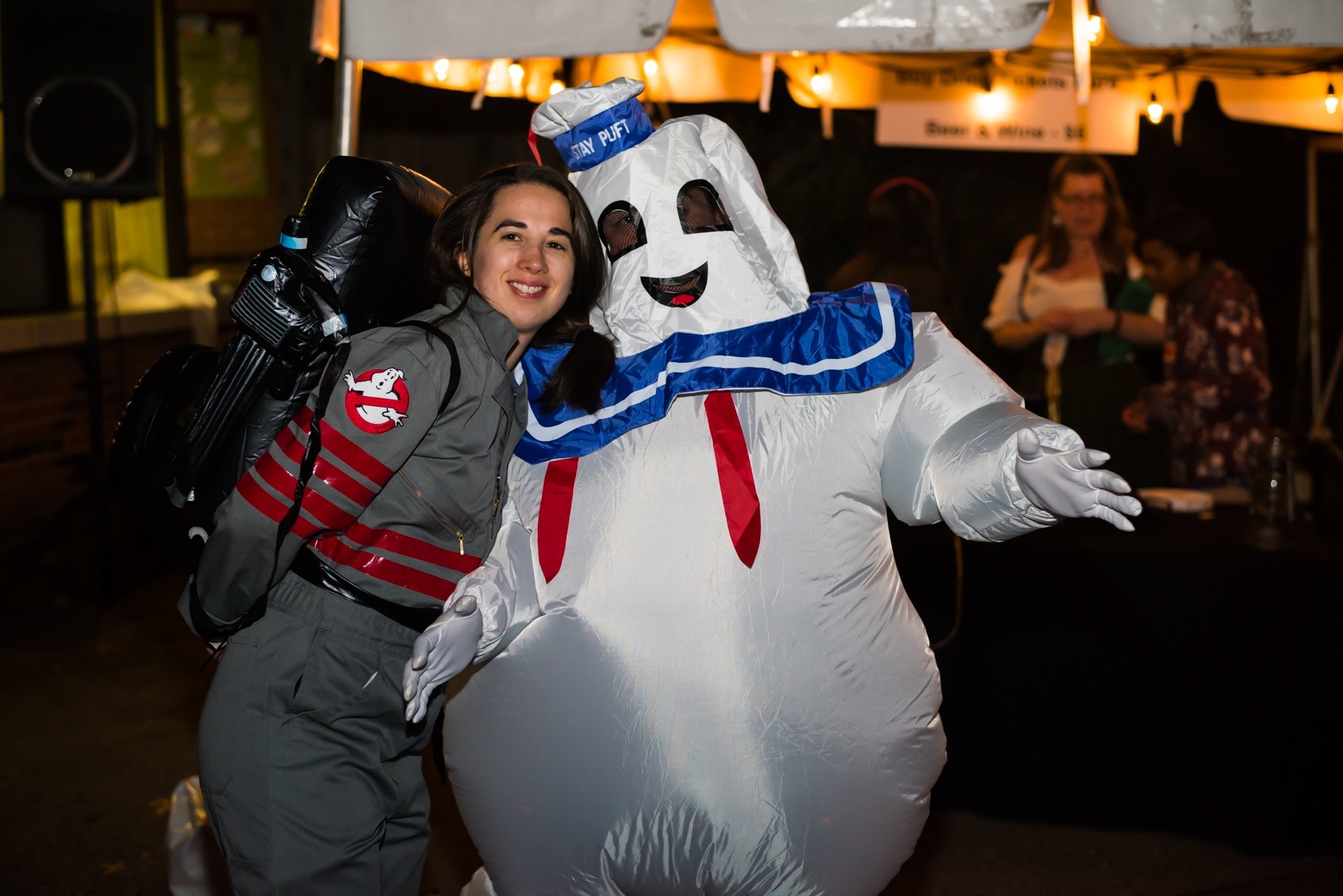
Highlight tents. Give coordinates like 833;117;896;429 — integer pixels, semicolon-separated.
311;1;1343;163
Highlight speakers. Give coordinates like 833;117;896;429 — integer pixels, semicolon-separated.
3;3;162;205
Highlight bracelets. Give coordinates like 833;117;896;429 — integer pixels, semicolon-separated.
1108;306;1124;336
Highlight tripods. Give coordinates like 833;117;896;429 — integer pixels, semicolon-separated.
13;197;163;596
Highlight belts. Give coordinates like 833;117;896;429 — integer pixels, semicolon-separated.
289;545;443;634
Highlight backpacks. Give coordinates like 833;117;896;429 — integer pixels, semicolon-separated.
102;326;241;560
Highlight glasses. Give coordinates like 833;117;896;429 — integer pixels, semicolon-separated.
1055;188;1111;206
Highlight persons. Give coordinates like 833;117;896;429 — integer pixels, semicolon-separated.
982;153;1166;492
397;75;1147;896
813;176;967;344
172;159;622;895
1116;205;1274;493
